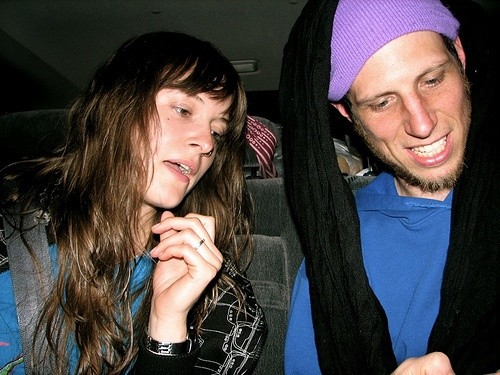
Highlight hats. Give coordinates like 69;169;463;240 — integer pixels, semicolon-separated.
328;0;459;103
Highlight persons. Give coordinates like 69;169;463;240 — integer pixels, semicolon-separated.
279;1;499;375
0;30;267;375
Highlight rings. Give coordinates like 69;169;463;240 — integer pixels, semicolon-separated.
194;238;206;251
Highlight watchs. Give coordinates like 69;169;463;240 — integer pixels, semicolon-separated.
141;324;199;357
